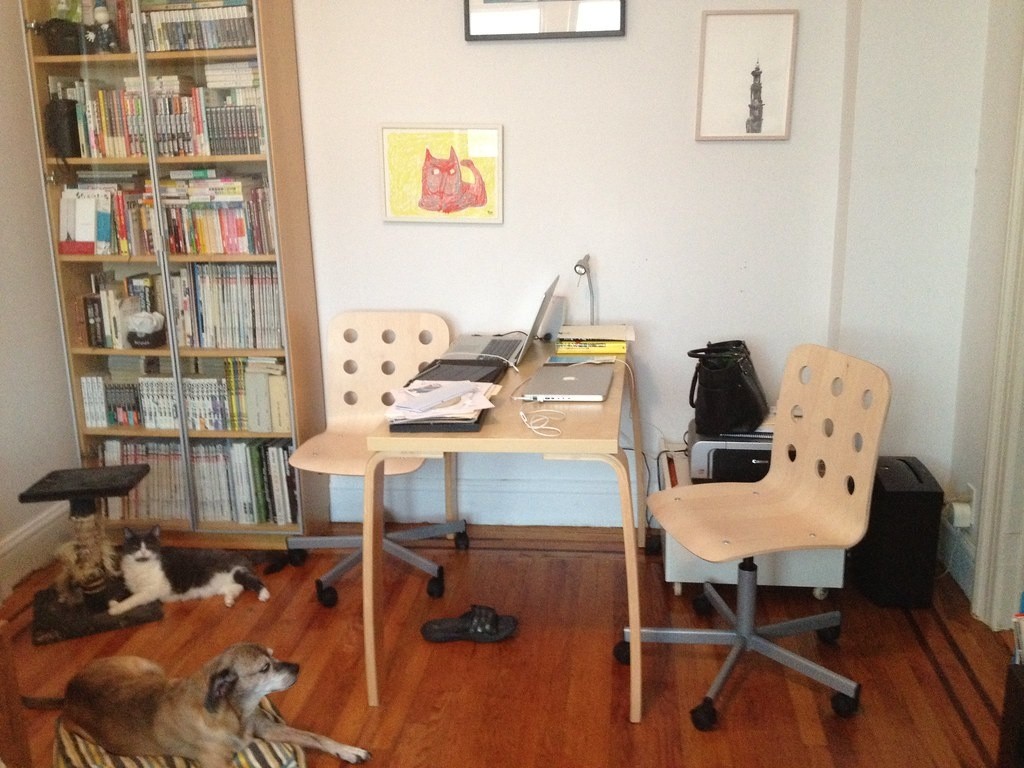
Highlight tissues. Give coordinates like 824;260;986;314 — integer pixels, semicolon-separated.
126;310;166;349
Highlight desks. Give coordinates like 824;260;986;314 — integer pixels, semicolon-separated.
654;437;847;599
361;337;644;724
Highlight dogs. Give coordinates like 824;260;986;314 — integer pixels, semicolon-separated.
19;641;372;768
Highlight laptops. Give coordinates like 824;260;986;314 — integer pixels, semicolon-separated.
436;275;560;367
521;365;616;402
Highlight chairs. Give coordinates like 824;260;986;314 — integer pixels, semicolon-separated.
611;340;895;732
284;308;470;606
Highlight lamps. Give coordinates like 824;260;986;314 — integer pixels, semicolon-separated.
574;255;595;327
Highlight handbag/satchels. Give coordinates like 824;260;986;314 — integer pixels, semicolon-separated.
686;339;770;438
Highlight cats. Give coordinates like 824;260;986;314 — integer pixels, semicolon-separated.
52;521;122;604
108;524;270;616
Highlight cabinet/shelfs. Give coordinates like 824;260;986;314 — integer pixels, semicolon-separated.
15;0;331;551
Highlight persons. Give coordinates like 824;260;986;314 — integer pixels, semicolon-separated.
85;0;118;52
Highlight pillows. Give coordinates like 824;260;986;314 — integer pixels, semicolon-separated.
52;695;304;768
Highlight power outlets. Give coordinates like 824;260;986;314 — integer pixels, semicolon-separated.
961;481;977;525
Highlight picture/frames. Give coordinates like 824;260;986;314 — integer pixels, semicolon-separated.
463;0;626;41
695;10;800;142
383;126;505;226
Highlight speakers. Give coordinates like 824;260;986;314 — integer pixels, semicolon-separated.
852;456;945;609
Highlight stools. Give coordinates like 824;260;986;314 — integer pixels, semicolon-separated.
16;463;163;645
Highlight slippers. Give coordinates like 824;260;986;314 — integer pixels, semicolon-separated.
421;604;517;644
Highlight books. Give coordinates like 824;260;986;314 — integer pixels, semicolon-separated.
58;0;300;526
556;324;635;354
